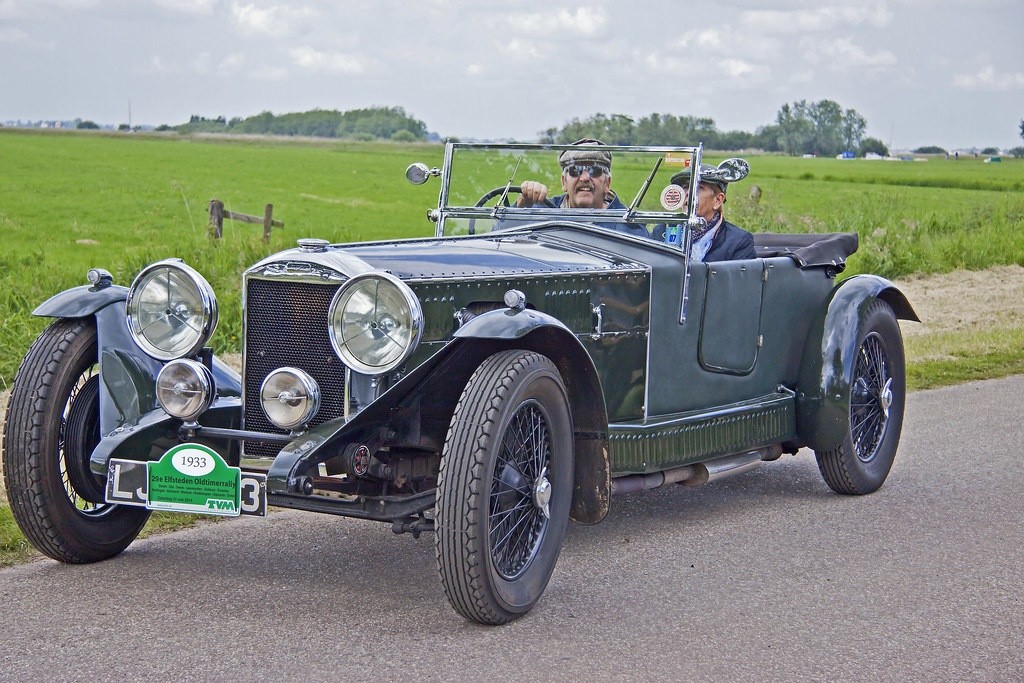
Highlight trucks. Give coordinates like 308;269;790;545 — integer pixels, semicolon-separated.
982;157;1002;166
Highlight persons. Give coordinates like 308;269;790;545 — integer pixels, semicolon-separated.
644;162;753;264
511;138;649;237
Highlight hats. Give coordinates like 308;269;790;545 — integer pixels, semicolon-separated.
670;157;727;204
558;138;611;171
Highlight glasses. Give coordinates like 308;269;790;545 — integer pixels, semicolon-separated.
564;165;609;178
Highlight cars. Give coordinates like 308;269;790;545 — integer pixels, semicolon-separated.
1;135;922;629
